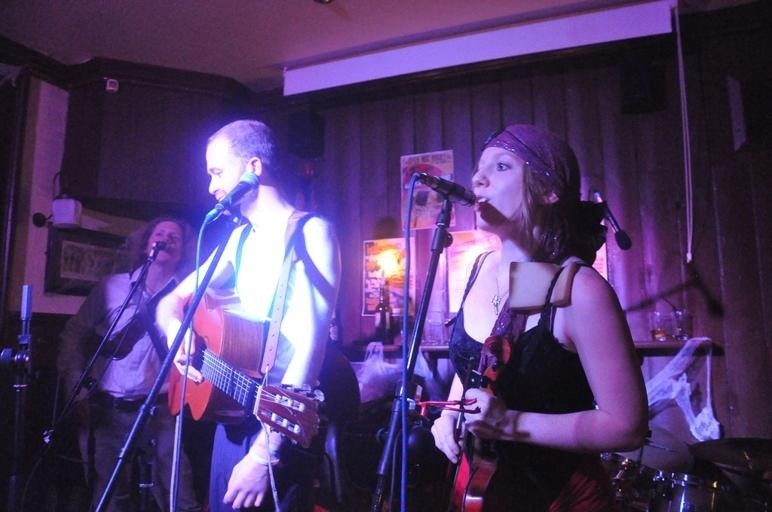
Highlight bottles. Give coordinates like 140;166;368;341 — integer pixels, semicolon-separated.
372;284;394;345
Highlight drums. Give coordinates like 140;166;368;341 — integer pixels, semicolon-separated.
599;454;665;512
662;472;734;512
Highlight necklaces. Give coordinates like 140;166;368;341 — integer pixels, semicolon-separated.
491;271;510;315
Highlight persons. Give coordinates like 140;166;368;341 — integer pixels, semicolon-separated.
61;214;207;512
431;123;649;512
156;120;343;512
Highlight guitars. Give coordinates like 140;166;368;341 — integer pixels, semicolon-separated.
168;286;331;457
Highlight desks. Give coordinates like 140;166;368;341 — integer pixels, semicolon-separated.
343;344;403;358
420;338;724;379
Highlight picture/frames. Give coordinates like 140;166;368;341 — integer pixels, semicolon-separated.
44;228;129;297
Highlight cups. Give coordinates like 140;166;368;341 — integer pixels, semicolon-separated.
645;310;693;342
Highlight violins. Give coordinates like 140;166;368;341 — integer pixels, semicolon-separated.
434;335;513;512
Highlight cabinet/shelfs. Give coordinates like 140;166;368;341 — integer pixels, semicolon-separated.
60;57;246;221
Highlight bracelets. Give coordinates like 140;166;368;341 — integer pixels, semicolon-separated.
249;447;280;467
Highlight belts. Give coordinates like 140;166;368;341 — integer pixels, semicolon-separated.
101;392;168;414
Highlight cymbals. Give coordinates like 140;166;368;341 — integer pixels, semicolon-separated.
689;437;772;485
640;440;678;454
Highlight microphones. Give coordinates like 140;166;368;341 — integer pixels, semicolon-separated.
593;190;631;250
204;171;260;224
153;240;169;251
414;172;476;208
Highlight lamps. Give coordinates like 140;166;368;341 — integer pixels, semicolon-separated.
33;193;83;228
